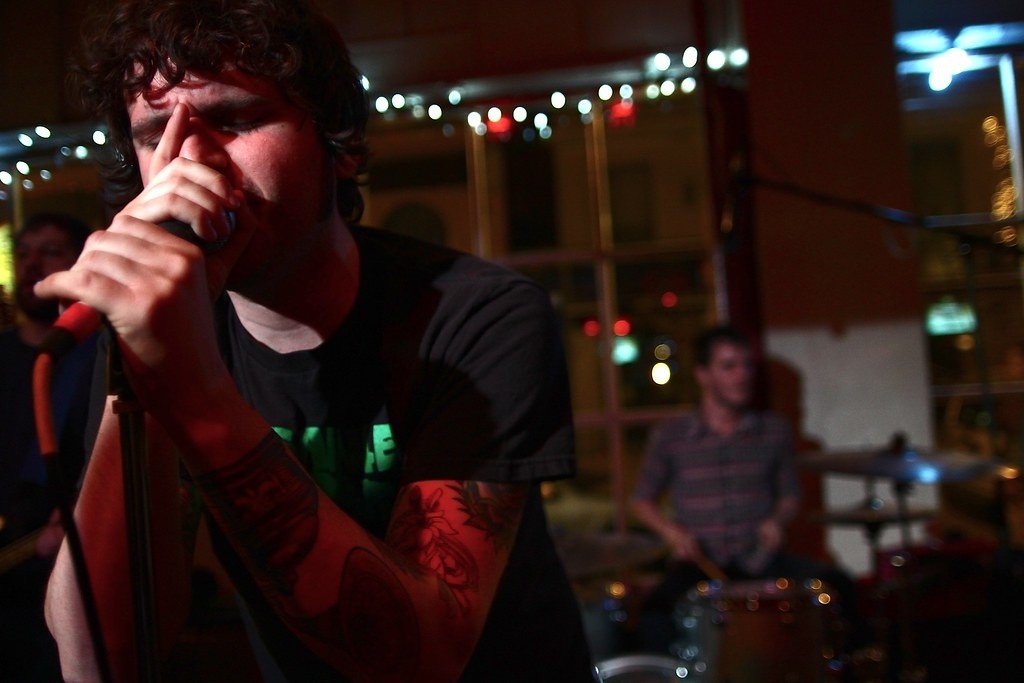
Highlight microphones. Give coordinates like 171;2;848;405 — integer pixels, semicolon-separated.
40;205;235;369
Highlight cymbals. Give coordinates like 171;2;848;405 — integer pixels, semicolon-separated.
804;442;982;487
819;504;933;529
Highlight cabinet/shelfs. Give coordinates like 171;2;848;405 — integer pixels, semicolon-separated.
353;76;727;547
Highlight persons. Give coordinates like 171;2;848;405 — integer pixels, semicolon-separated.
0;208;97;581
42;1;601;683
621;322;862;656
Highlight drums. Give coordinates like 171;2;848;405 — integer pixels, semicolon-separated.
679;571;834;681
